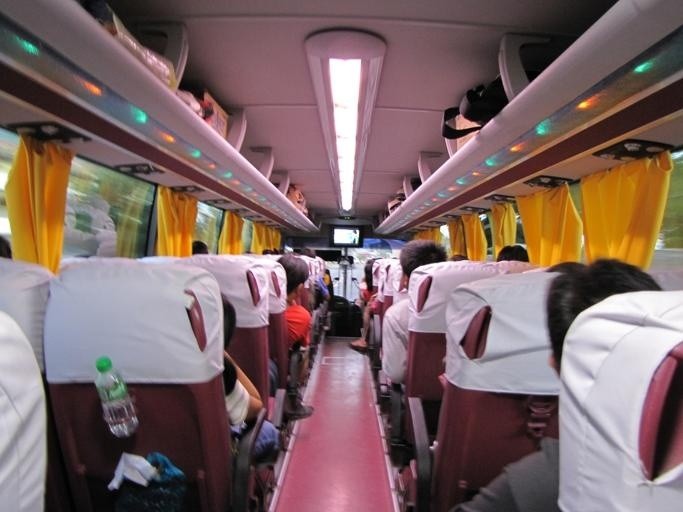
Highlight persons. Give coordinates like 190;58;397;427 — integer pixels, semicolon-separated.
449;258;665;511
0;236;13;258
193;238;590;465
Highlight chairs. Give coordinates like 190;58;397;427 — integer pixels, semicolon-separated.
139;252;331;451
395;268;573;511
41;254;276;511
556;288;683;512
0;310;53;510
1;255;57;458
365;253;540;459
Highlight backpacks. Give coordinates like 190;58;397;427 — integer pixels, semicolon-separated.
441;71;539;138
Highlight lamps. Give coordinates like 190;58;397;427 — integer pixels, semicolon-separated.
303;29;386;216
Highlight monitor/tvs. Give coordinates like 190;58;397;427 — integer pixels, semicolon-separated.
329;224;363;248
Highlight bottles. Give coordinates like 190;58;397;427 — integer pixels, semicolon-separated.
92;356;139;441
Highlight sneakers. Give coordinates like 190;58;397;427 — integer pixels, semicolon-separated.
351;339;367;348
285;403;314;420
